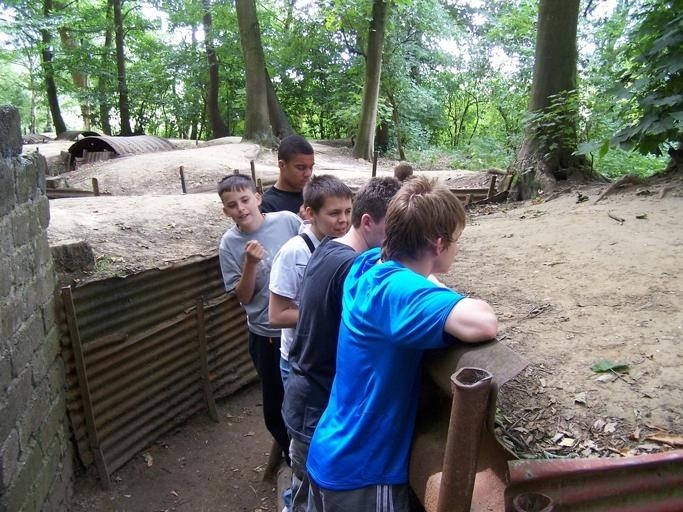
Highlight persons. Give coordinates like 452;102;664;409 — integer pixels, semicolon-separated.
280;176;402;512
216;174;306;471
258;134;315;216
306;172;497;512
267;174;353;512
394;160;413;188
